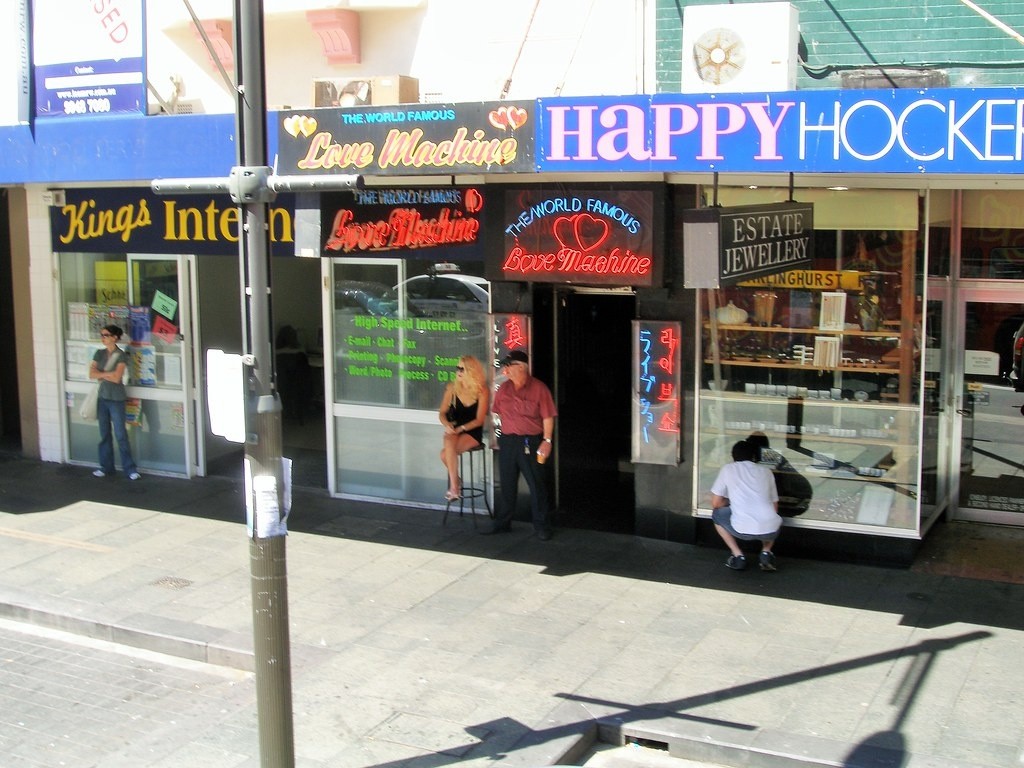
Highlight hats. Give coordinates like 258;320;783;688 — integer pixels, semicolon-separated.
498;350;528;364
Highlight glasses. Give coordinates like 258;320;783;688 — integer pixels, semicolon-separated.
99;333;113;337
502;362;520;367
455;365;465;371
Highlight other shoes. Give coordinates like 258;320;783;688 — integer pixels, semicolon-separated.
537;528;552;541
93;470;106;477
479;525;511;535
444;476;463;501
128;472;141;480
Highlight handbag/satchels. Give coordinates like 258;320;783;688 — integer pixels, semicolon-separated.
80;387;99;422
445;388;456;423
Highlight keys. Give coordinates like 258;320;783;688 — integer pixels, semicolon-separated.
524;444;530;455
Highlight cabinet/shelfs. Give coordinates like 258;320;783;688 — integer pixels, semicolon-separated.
698;230;917;527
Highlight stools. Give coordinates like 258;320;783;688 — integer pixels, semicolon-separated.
441;443;493;529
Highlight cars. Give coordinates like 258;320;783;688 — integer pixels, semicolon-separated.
334;279;458;367
380;262;489;340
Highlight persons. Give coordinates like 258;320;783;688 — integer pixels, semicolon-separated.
89;324;142;480
474;350;559;541
745;430;813;518
439;356;490;502
710;440;783;571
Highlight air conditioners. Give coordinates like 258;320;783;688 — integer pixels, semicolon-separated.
679;3;802;93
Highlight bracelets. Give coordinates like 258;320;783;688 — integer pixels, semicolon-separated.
541;438;553;443
460;425;466;433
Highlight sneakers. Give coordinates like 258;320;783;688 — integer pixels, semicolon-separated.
723;554;746;569
759;551;776;570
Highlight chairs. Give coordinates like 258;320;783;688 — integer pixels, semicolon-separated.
276;323;326;426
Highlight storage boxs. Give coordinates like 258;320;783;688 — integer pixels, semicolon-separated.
372;76;419;106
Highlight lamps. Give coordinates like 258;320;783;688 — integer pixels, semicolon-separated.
338;81;372;106
